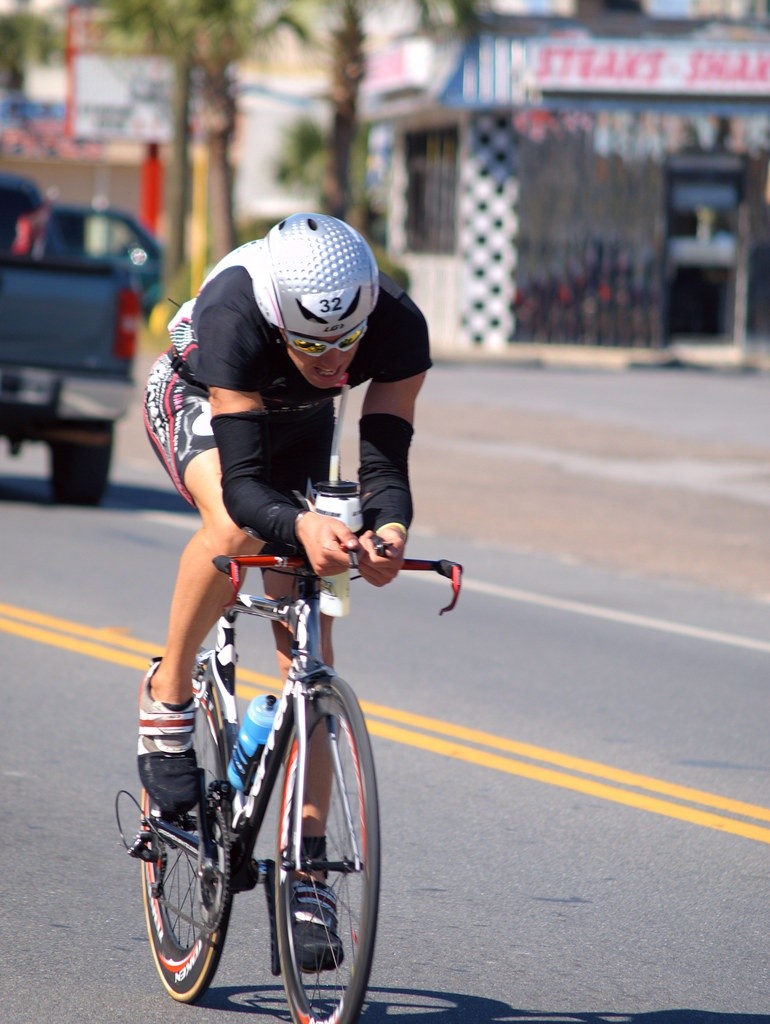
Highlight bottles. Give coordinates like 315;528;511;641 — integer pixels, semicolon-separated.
226;694;279;794
315;479;363;618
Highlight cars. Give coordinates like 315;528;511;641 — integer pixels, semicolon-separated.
47;200;164;319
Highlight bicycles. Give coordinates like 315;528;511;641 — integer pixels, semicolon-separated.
113;554;464;1023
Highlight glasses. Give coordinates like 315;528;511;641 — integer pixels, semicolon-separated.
280;319;367;356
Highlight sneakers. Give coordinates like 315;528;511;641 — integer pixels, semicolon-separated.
292;878;344;973
138;656;201;816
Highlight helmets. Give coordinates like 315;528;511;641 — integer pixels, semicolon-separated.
262;212;379;336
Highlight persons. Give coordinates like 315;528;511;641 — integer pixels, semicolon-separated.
139;211;431;816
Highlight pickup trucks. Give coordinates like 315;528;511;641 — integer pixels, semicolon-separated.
0;171;143;507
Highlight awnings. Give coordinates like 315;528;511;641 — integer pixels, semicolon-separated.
436;28;770;115
